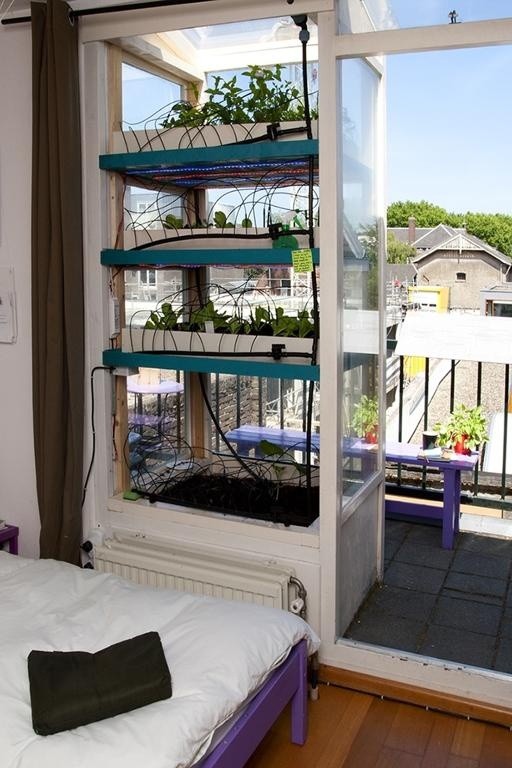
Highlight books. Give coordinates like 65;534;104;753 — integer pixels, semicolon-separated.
350;437;378;453
417;447;450;462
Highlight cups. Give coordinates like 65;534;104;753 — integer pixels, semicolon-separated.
422;430;439;450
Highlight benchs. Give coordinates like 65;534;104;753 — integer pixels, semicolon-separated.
220;425;479;551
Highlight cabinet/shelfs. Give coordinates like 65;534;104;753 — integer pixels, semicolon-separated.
75;0;388;637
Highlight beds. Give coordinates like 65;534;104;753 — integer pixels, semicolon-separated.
0;550;311;768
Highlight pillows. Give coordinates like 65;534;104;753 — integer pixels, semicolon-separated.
22;629;169;736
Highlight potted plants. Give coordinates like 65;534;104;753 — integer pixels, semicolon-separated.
431;403;489;455
350;396;377;446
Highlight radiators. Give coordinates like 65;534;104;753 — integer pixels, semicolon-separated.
85;543;305;616
